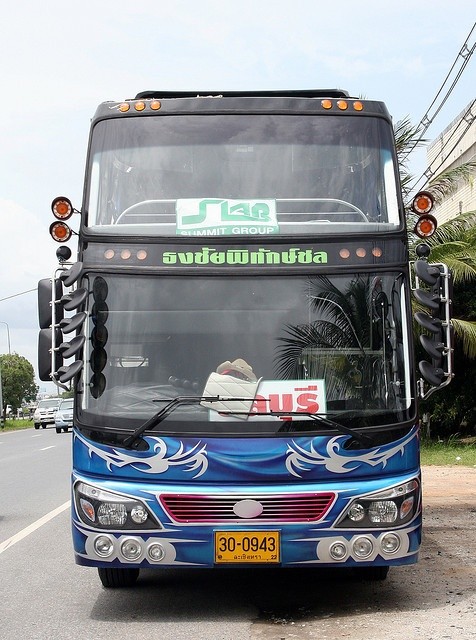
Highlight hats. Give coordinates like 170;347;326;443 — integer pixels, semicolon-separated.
216;358;257;383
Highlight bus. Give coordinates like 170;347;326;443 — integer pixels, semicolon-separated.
37;89;455;588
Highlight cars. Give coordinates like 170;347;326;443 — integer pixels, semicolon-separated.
33;398;64;429
55;398;74;433
